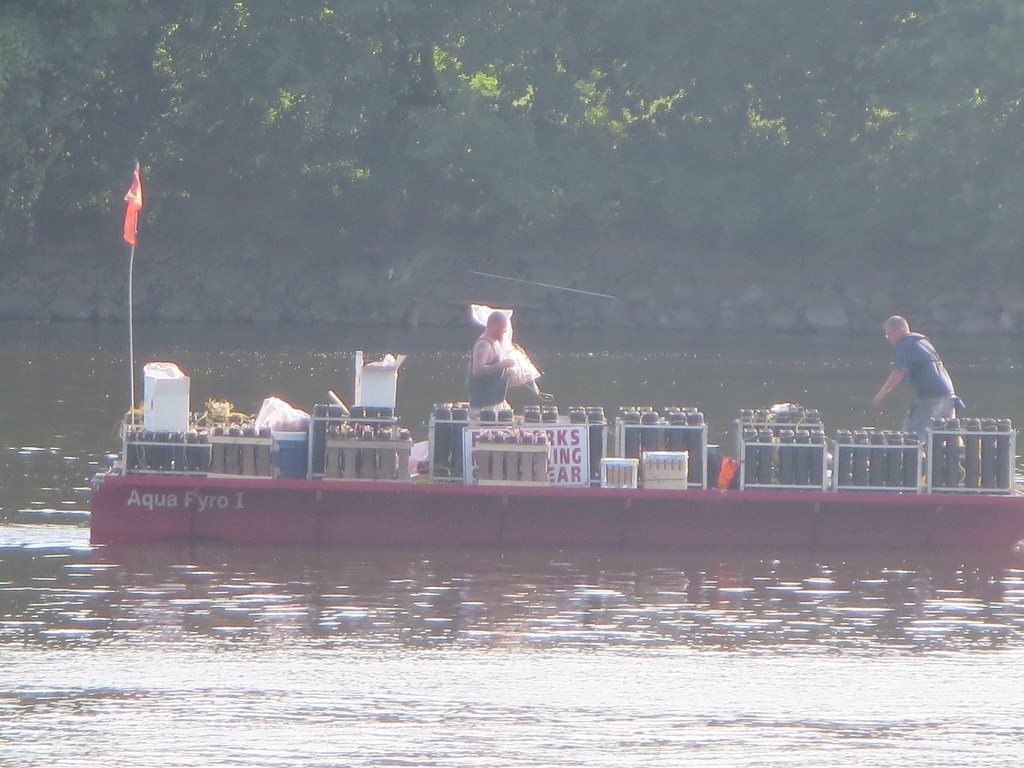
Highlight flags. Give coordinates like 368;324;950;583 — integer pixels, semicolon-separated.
122;169;143;246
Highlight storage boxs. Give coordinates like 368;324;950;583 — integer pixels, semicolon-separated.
642;451;688;490
271;431;307;479
600;457;640;489
476;442;551;487
355;350;407;409
207;434;275;480
143;374;190;433
323;438;413;483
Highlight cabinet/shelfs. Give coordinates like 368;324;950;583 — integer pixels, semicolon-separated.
121;424;213;477
615;416;708;490
831;439;922;495
428;412;519;483
734;419;827;493
307;415;398;481
925;427;1017;495
520;417;608;486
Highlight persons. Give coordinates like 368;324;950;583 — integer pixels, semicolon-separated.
872;316;965;490
466;312;521;427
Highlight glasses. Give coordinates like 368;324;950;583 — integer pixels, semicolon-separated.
885;327;900;339
